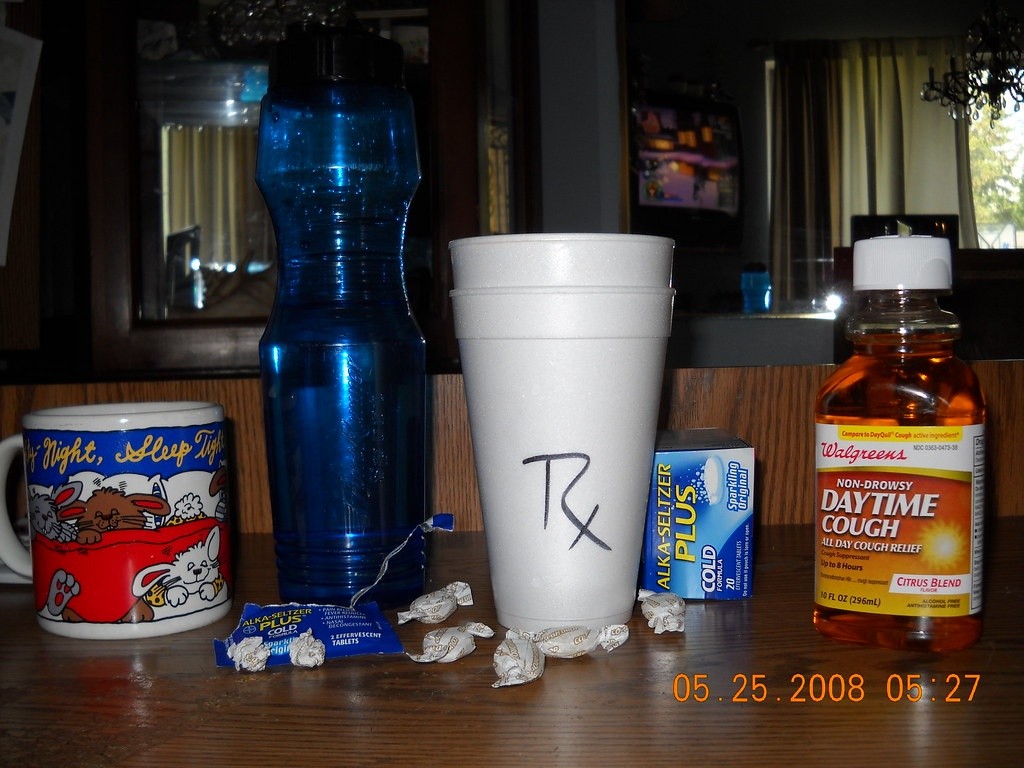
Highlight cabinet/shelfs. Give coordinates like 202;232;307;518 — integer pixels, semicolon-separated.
537;0;852;368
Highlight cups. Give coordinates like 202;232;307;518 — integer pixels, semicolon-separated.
448;233;675;630
0;402;232;640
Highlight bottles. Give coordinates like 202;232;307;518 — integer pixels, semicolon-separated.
735;262;773;315
256;32;426;610
814;235;986;653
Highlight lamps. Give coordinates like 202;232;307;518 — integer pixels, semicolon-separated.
920;0;1024;128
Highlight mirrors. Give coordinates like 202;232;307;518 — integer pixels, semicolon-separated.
70;1;483;373
